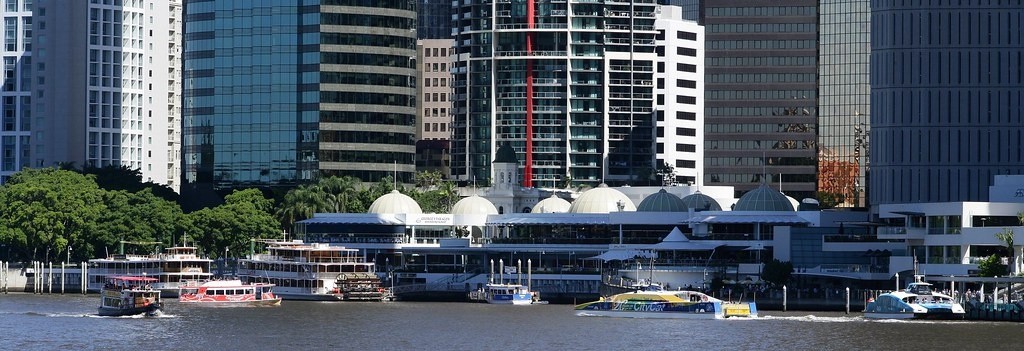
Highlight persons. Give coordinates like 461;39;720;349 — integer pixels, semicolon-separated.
685;283;692;289
705;285;827;298
665;282;670;291
934;287;993;304
104;280;154;291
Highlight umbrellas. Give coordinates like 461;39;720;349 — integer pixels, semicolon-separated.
697;279;774;285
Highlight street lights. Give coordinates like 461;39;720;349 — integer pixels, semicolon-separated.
568;247;575;275
225;246;230;267
703;268;709;291
636;261;643;284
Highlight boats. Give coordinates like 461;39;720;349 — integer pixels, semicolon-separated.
326;271;398;302
178;275;283;309
486;258;533;306
860;248;967;321
572;289;759;320
97;275;165;317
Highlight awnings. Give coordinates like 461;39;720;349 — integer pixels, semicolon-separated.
294;216;406;225
